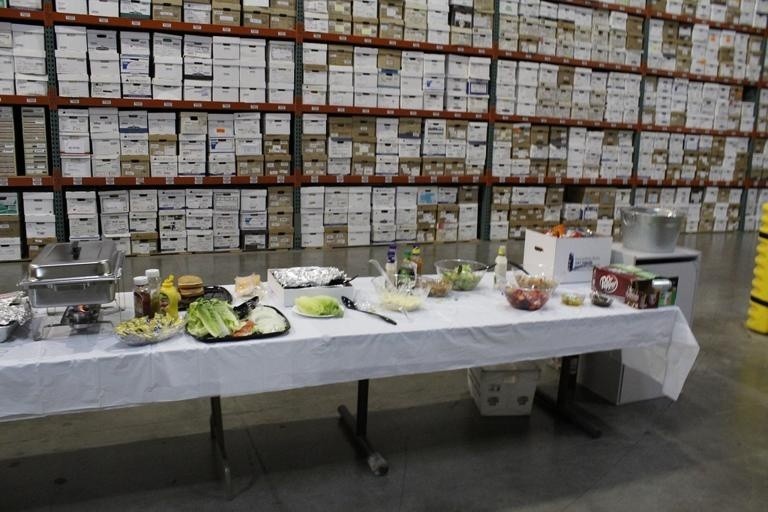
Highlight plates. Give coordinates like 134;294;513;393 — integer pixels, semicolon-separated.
177;286;233;311
114;315;187;347
292;302;344;318
185;303;290;343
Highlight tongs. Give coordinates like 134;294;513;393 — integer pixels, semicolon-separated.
234;294;260;318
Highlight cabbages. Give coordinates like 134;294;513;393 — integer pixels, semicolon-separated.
295;295;343;318
187;297;241;337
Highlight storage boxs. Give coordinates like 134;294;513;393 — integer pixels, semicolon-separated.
0;18;50;98
0;103;50;178
491;121;635;180
1;185;58;263
634;187;743;234
298;184;481;250
0;0;43;11
494;58;642;125
744;186;768;234
303;0;494;48
646;16;763;83
63;185;295;259
749;137;768;180
637;131;750;187
489;184;632;241
522;224;613;286
641;73;758;133
54;0;297;32
300;40;492;113
651;0;768;28
57;106;292;180
756;87;768;133
468;361;541;416
299;110;489;179
52;21;297;104
498;0;644;69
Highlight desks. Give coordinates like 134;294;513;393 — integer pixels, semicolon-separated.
0;270;698;501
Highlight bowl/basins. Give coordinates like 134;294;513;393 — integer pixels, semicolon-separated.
562;288;587;305
620;205;686;256
372;273;433;313
434;258;488;291
515;274;560;296
589;289;614;307
420;275;453;298
502;287;554;310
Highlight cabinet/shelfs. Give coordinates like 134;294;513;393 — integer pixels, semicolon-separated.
577;242;704;406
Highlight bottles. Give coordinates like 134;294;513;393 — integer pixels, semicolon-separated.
132;275;151;318
411;247;423;276
494;245;508;280
158;274;179;320
145;268;162;313
400;249;415;280
385;244;398;278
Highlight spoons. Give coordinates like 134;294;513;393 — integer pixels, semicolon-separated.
341;295;397;325
